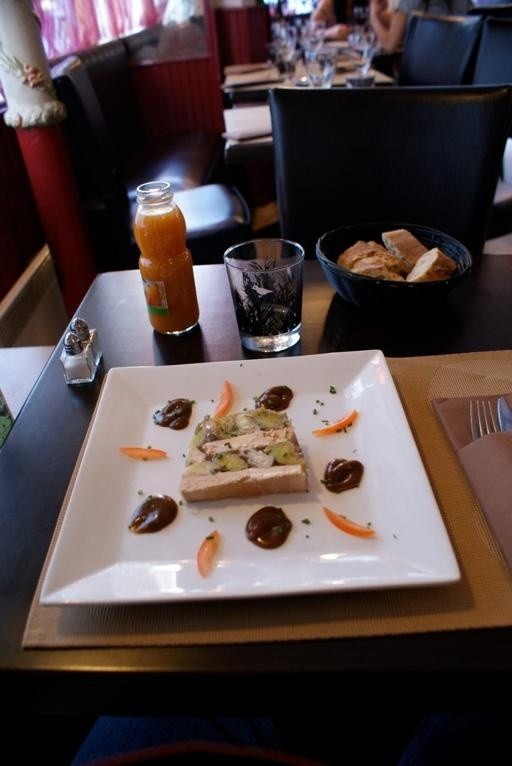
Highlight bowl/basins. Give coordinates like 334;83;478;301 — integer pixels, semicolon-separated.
314;218;474;325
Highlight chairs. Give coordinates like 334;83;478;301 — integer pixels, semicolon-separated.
396;10;485;86
268;83;512;262
49;56;256;270
473;16;512;83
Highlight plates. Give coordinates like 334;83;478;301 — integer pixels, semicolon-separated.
37;349;463;608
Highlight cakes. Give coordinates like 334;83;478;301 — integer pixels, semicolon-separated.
179;410;310;503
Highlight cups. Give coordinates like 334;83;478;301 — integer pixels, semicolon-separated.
224;233;307;361
270;22;302;84
302;46;339;90
346;27;381;84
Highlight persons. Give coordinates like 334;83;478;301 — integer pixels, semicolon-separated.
310;0;373;42
363;0;410;88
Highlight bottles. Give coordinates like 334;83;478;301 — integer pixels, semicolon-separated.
70;318;101;366
133;180;200;338
60;332;96;384
